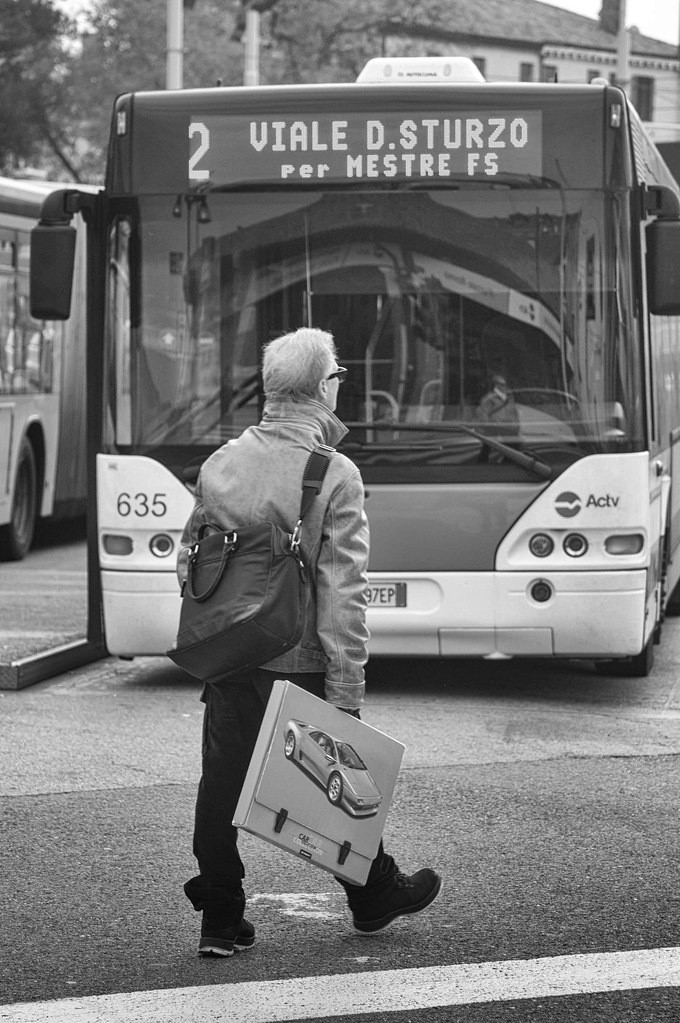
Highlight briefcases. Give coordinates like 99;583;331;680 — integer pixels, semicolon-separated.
232;678;405;889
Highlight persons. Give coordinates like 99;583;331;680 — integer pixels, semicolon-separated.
167;329;442;957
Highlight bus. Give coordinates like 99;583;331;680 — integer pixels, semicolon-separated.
29;56;680;678
1;169;133;562
175;175;620;448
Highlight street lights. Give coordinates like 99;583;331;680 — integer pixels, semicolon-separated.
165;180;214;452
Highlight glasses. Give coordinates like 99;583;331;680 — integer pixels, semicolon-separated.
328;367;348;384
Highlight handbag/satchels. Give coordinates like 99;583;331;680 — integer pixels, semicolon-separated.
167;444;336;684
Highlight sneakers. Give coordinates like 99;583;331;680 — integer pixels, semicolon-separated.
354;867;443;935
195;914;257;956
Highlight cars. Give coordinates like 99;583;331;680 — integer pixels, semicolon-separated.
283;717;384;817
